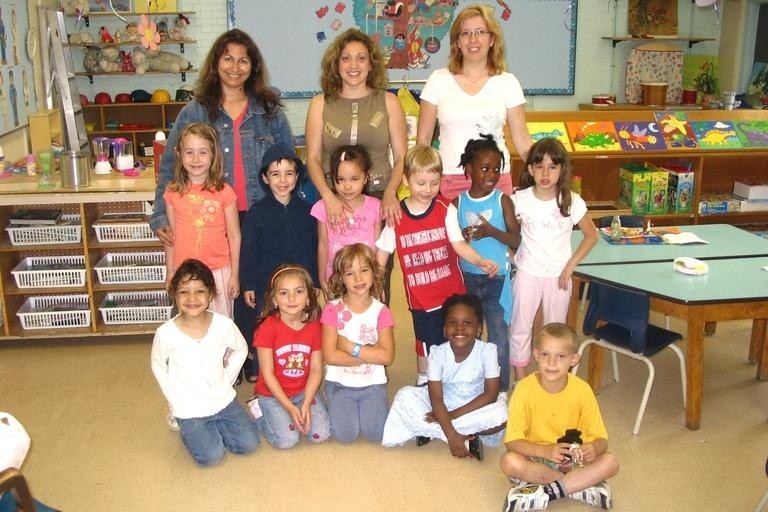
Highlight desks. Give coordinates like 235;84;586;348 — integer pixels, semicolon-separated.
566;222;767;336
567;254;768;431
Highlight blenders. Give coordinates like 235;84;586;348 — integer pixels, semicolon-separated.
92;137;113;175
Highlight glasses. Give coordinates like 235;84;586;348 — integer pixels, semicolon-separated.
458;30;490;40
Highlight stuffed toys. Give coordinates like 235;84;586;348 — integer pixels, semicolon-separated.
59;0;190;76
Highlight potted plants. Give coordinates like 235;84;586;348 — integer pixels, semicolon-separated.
694;63;718;106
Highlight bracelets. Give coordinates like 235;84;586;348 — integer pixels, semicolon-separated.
352;343;361;359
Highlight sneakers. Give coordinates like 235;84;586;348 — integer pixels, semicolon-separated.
247;396;263;419
167;403;181;431
569;480;611;509
503;480;550;512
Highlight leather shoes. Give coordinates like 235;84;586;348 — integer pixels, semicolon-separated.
416;435;431;445
469;434;482;460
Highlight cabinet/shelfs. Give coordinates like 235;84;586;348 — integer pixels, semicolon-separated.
57;3;198;163
504;107;767;230
0;157;172;341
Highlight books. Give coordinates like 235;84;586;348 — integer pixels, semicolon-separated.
8;208;159;285
698;177;768;216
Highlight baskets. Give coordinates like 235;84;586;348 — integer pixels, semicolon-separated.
15;295;91;330
11;255;87;289
5;214;82;245
92;213;162;242
94;252;167;284
98;291;175;325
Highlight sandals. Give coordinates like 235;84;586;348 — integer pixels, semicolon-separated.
235;357;257;386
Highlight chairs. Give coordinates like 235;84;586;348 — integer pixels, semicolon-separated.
570;279;688;433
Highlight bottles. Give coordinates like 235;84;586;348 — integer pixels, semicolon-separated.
26;155;37;177
610;216;621;242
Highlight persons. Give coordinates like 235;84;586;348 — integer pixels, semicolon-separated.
414;2;535;202
150;258;262;468
382;293;509;461
451;132;522;403
509;136;600;393
320;243;396;444
500;322;619;512
245;263;334;450
304;27;409;309
163;123;241;321
374;144;501;388
148;28;304;386
0;6;31;130
310;145;389;304
238;140;319;377
